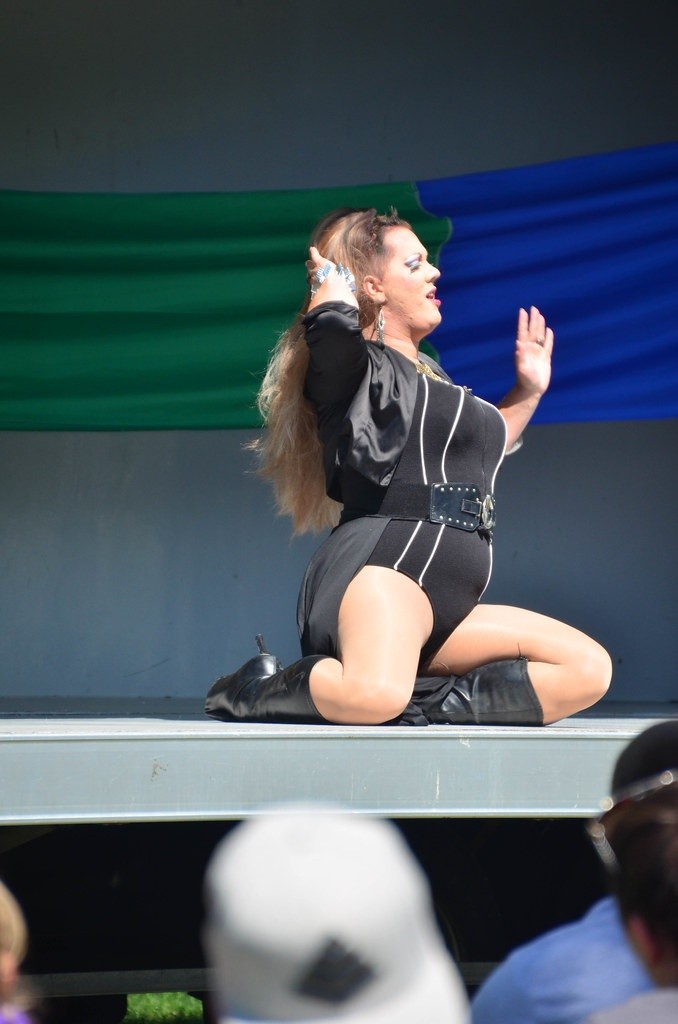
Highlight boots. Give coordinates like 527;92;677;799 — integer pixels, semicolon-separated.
204;652;342;725
380;657;544;726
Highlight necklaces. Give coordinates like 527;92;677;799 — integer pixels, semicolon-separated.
415;359;441;381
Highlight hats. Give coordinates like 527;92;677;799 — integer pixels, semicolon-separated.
201;820;473;1024
612;719;678;805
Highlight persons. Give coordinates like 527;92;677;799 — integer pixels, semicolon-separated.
205;206;613;726
0;884;40;1019
206;810;470;1024
469;724;678;1021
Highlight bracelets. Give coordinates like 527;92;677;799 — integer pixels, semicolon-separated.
308;263;357;300
